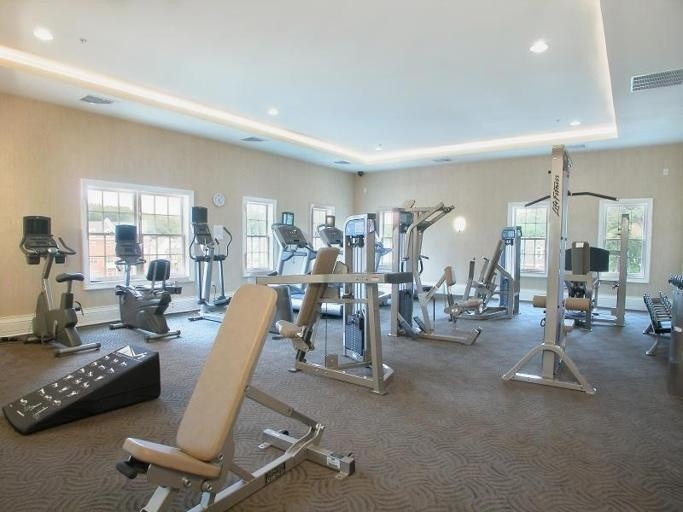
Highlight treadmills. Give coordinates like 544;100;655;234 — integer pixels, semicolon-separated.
268;224;434;317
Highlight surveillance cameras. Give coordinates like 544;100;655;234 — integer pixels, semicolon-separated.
358;171;363;176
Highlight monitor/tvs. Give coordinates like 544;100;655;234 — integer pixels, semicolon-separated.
23;216;51;240
192;207;207;223
325;215;335;227
116;225;136;242
282;212;294;226
